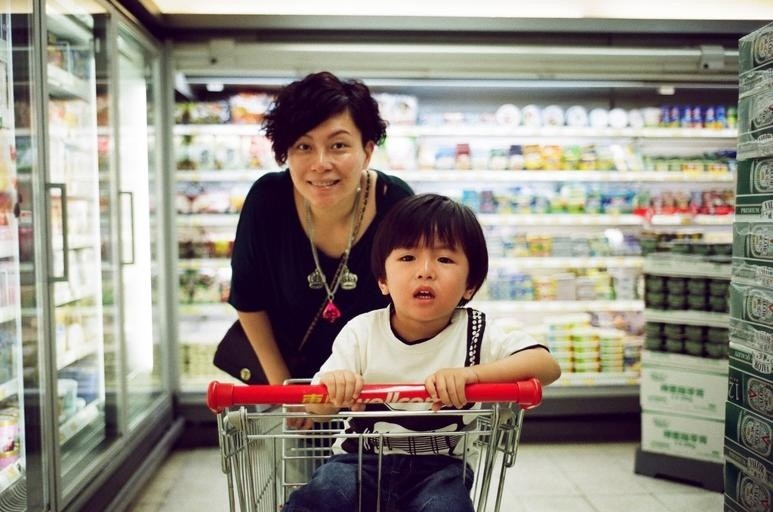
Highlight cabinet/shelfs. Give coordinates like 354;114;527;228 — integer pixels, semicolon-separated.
0;69;739;494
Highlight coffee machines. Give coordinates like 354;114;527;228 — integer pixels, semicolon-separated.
0;0;178;510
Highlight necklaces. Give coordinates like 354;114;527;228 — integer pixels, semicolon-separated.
304;194;361;324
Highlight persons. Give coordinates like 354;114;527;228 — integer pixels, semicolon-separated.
227;72;417;490
279;194;561;512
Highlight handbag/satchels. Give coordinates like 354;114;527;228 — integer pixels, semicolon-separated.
214;315;310;386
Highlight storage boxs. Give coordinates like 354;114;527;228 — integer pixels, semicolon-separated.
723;21;773;512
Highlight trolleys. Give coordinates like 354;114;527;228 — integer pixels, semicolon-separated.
208;378;543;512
